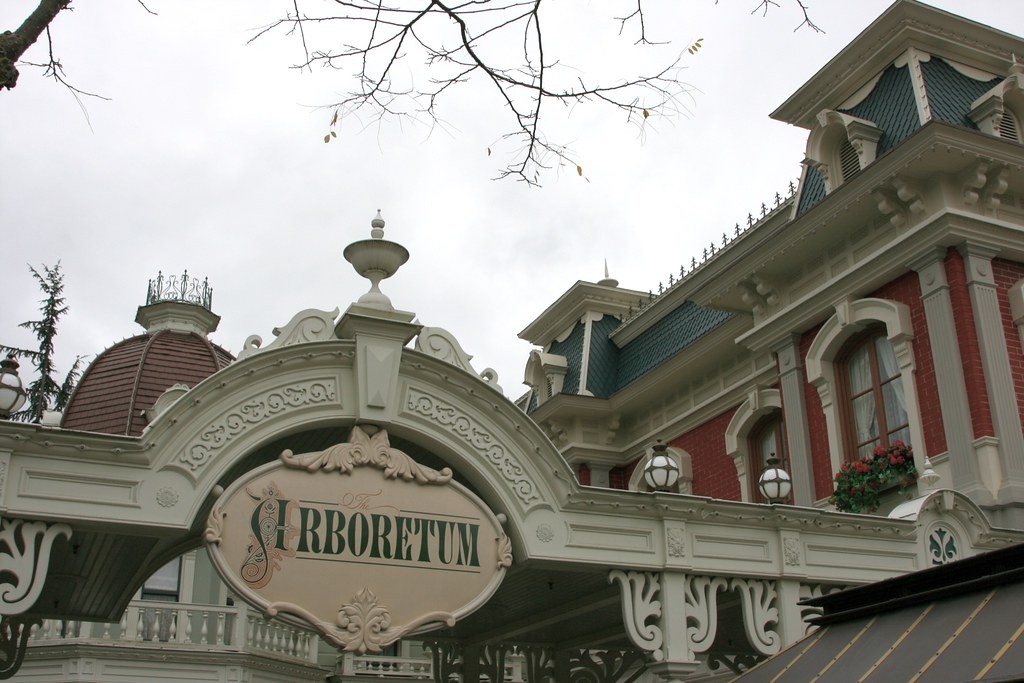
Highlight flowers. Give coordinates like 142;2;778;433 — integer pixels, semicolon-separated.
829;441;922;516
